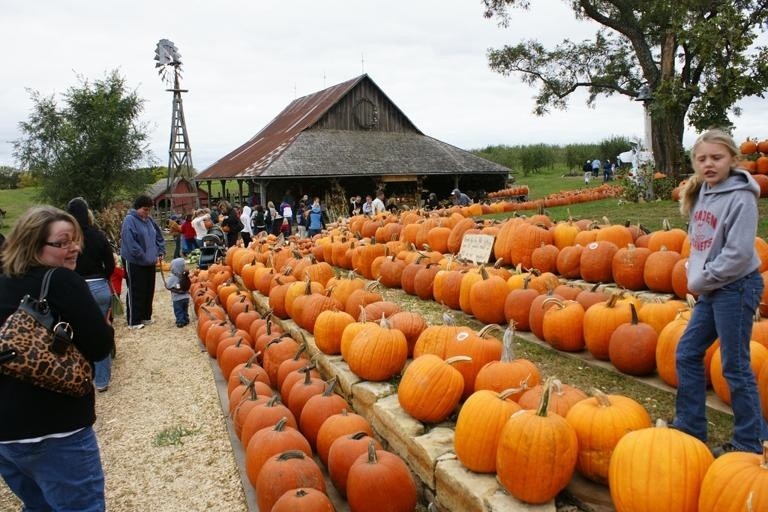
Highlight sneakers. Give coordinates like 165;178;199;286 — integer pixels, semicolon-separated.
128;324;144;330
714;443;763;457
141;319;156;324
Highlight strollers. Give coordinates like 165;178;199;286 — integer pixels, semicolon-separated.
198;225;228;270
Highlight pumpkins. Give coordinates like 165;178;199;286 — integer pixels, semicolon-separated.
189;139;768;512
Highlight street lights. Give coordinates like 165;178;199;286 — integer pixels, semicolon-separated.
633;84;657;199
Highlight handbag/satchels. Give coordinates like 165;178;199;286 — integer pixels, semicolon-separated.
232;218;244;233
0;293;95;399
111;293;126;319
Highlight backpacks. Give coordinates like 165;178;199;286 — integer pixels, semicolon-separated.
169;271;191;294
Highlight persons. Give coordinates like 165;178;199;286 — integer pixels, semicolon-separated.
603;159;612;181
0;203;113;512
165;258;191;328
119;195;166;329
611;162;616;175
66;196;116;392
591;158;601;179
669;127;768;457
110;252;126;299
583;159;592;187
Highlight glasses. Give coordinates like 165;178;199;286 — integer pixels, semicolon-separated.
45;237;80;249
